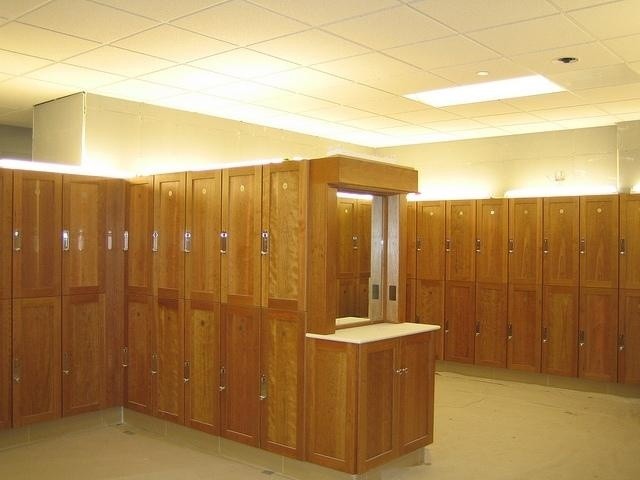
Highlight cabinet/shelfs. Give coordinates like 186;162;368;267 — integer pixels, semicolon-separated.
0;156;640;480
